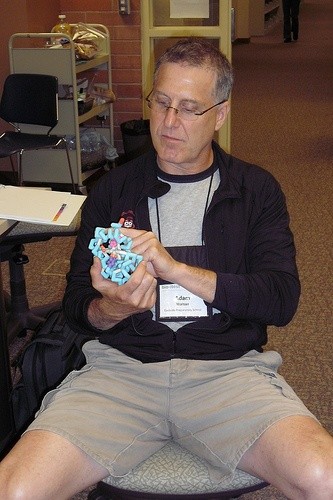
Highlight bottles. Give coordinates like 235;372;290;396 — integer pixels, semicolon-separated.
54;14;73;49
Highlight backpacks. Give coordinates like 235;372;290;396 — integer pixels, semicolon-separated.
9;309;95;439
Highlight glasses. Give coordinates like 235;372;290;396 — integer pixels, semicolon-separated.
145;88;225;121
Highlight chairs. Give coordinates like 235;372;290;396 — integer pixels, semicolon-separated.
0;208;83;346
87;445;270;500
0;73;77;196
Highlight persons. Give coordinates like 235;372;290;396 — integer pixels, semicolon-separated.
282;0;301;42
0;37;333;500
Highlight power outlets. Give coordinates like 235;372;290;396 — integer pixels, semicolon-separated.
118;0;130;15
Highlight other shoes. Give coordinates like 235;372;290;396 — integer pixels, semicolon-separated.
285;38;291;42
293;30;298;40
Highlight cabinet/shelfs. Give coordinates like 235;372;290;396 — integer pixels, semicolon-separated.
250;0;283;37
